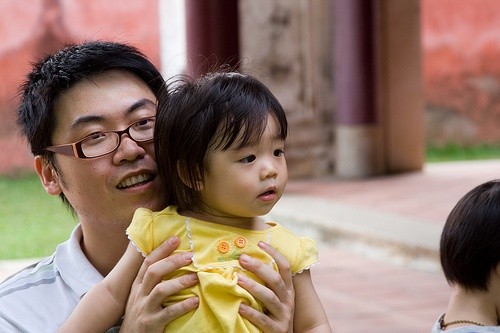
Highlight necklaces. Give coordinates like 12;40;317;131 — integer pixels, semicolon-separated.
439;314;485;330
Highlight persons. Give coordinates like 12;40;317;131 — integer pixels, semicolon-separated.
431;180;500;332
55;70;334;333
0;39;296;333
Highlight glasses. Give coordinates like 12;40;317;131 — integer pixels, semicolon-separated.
41;115;156;161
